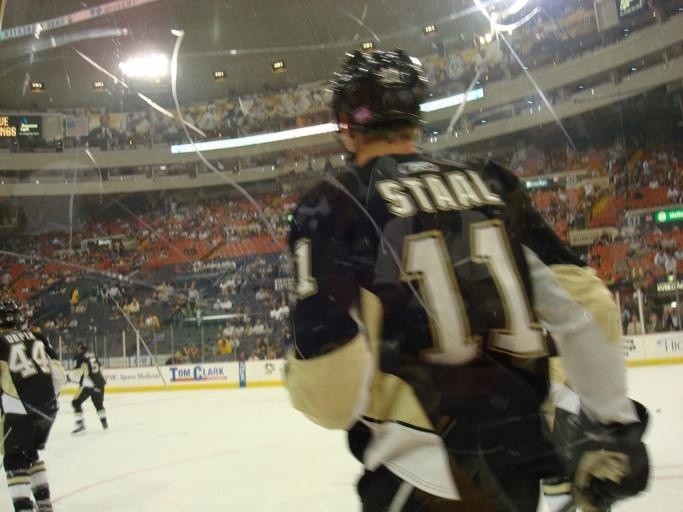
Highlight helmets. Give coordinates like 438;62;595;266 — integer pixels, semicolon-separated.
0;297;20;328
328;45;431;127
76;341;87;353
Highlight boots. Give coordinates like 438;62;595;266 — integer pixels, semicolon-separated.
72;420;84;433
100;419;109;430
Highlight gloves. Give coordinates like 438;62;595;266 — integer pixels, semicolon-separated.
572;400;650;511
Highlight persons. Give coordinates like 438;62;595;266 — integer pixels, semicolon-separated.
0;297;69;511
277;47;651;512
2;3;681;365
64;340;111;435
